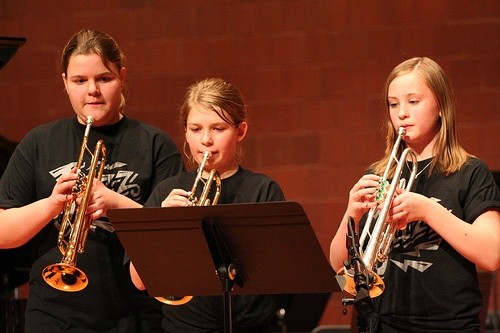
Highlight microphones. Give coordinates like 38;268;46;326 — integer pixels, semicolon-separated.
346;217;363;277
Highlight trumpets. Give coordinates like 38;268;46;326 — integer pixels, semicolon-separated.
153;150;222;306
336;128;418;299
43;115;107;293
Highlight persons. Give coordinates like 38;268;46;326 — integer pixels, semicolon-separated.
328;57;500;333
123;77;288;333
0;27;188;332
